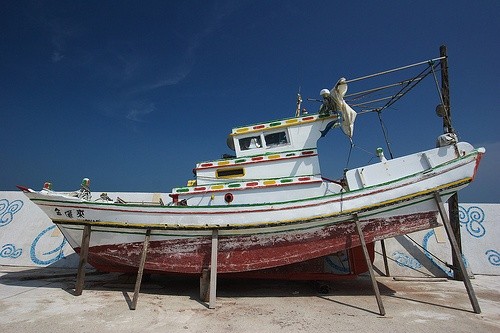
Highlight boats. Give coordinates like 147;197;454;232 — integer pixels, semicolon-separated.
16;54;485;284
